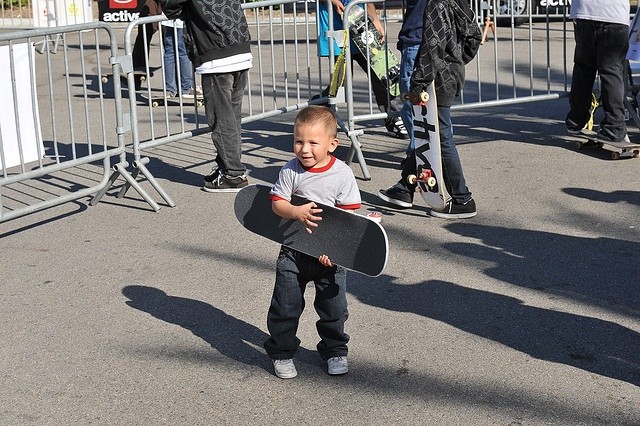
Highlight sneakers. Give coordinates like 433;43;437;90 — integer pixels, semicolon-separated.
378;181;415;207
385;122;409;139
272;357;297;380
183;87;204;99
565;119;581;134
429;195;478;219
165;89;176;98
204;173;248;192
599;128;614;141
203;166;223;182
327;357;349;375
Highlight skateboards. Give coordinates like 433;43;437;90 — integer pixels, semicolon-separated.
340;4;401;96
234;184;388;278
88;64;153;84
401;79;445;210
141;91;205;108
569;128;640;159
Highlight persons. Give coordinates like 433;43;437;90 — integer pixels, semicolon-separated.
132;0;252;192
566;1;630;142
378;1;482;218
307;1;412;137
262;103;362;380
